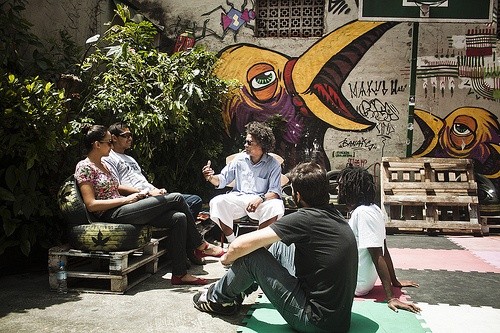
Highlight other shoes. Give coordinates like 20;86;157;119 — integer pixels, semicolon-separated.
189;255;206;265
195;242;225;257
171;272;208;285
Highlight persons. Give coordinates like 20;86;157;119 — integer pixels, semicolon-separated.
74;123;224;285
192;162;359;333
202;120;285;245
101;123;207;266
268;166;422;315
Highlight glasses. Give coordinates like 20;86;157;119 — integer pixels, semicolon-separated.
92;139;114;147
242;139;257;146
117;131;133;138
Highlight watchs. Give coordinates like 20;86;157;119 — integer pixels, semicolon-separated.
258;193;267;203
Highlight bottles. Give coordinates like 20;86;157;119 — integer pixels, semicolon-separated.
58;261;68;296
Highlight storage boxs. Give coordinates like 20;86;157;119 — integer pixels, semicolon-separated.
47;219;216;295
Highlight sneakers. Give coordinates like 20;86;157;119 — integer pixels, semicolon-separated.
192;290;246;316
